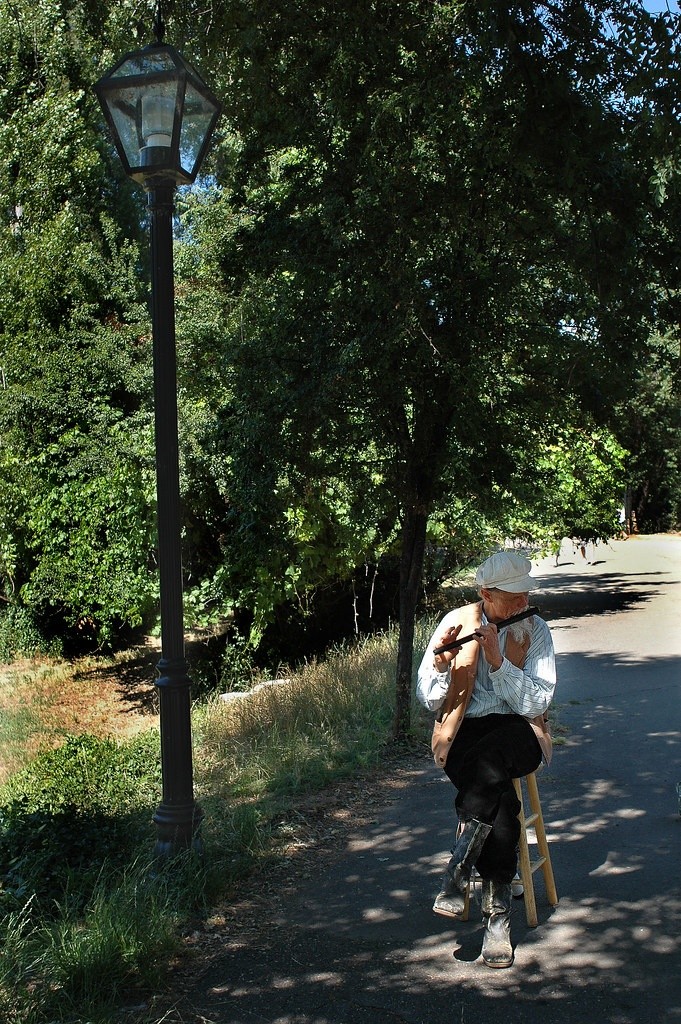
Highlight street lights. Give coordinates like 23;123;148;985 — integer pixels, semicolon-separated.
87;40;232;906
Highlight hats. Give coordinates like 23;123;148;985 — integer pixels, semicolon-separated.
475;552;541;593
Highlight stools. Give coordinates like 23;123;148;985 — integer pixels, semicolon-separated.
452;767;560;935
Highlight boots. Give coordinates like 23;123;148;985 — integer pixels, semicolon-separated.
432;819;492;920
481;878;514;968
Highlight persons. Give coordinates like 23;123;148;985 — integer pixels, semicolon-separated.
554;536;591;567
613;505;629;541
415;552;556;968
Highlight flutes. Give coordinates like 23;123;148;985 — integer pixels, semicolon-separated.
433;606;541;656
